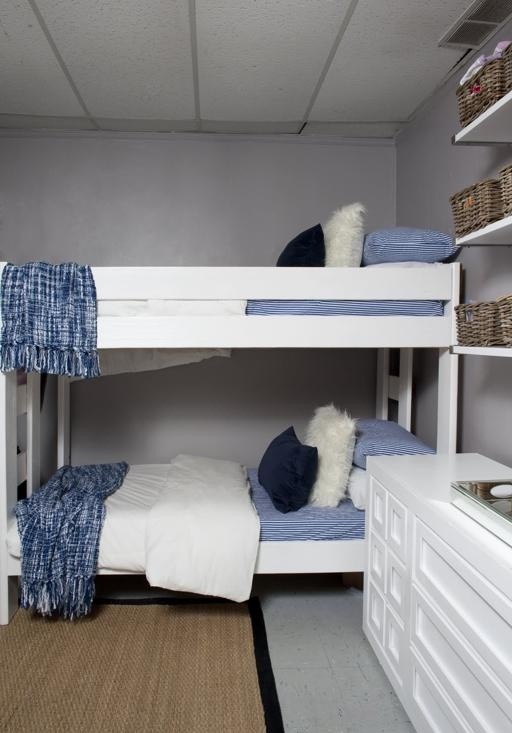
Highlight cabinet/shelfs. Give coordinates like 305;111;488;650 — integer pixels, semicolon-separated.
361;451;511;730
449;86;512;361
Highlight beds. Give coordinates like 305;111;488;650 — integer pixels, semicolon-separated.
0;260;463;626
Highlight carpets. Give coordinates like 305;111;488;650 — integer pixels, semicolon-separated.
0;590;286;733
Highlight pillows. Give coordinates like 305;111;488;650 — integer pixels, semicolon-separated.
274;200;462;267
257;402;436;515
